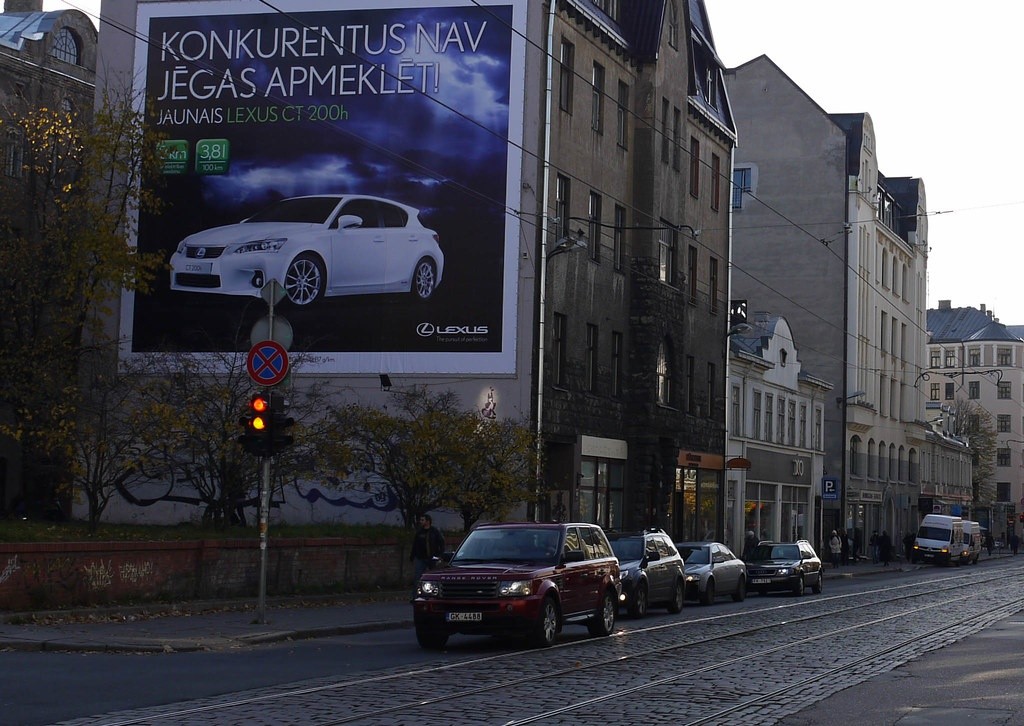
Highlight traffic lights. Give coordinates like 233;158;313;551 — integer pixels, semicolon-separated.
271;413;294;453
1020;515;1023;523
238;412;253;451
250;394;272;456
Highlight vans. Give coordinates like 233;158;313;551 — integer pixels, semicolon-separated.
961;520;981;565
911;514;964;567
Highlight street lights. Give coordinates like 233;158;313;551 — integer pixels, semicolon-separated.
1005;440;1024;451
527;237;588;524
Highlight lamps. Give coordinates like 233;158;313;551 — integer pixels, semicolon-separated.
378;374;422;397
724;323;753;340
547;236;587;265
836;391;866;404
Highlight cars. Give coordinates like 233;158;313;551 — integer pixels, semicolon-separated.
675;543;747;606
993;538;1004;549
170;194;443;311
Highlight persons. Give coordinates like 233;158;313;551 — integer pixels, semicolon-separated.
829;529;842;569
742;531;760;561
410;514;445;603
843;531;856;565
544;534;565;555
1011;533;1021;556
799;536;813;548
903;532;917;562
870;530;892;566
985;532;995;556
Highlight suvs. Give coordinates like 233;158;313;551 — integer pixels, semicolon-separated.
604;527;686;618
745;540;823;596
413;523;622;648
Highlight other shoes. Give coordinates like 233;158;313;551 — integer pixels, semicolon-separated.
410;599;413;603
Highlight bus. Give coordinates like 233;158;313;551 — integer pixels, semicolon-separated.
980;528;989;546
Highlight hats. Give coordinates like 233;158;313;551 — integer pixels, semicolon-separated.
748;531;754;536
832;530;837;535
